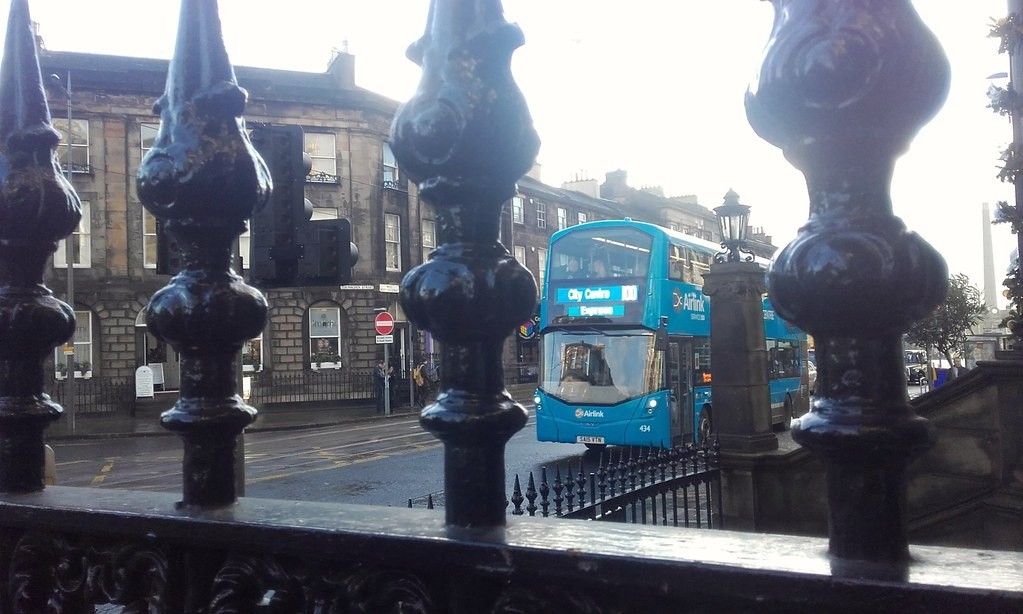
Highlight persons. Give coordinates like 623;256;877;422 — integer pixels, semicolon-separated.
415;358;430;408
388;367;399;412
919;368;926;381
948;357;970;380
590;257;608;277
374;359;388;412
561;257;585;279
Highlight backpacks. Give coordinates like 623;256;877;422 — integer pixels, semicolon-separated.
413;364;425;386
429;365;439;382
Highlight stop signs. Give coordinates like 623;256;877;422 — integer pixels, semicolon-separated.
375;312;394;335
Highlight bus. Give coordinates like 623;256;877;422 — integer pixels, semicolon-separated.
533;216;808;449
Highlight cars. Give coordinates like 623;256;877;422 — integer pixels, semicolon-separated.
905;348;928;382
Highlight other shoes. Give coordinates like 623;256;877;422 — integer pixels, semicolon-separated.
417;400;425;408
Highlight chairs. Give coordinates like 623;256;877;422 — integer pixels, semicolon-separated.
566;262;804;397
